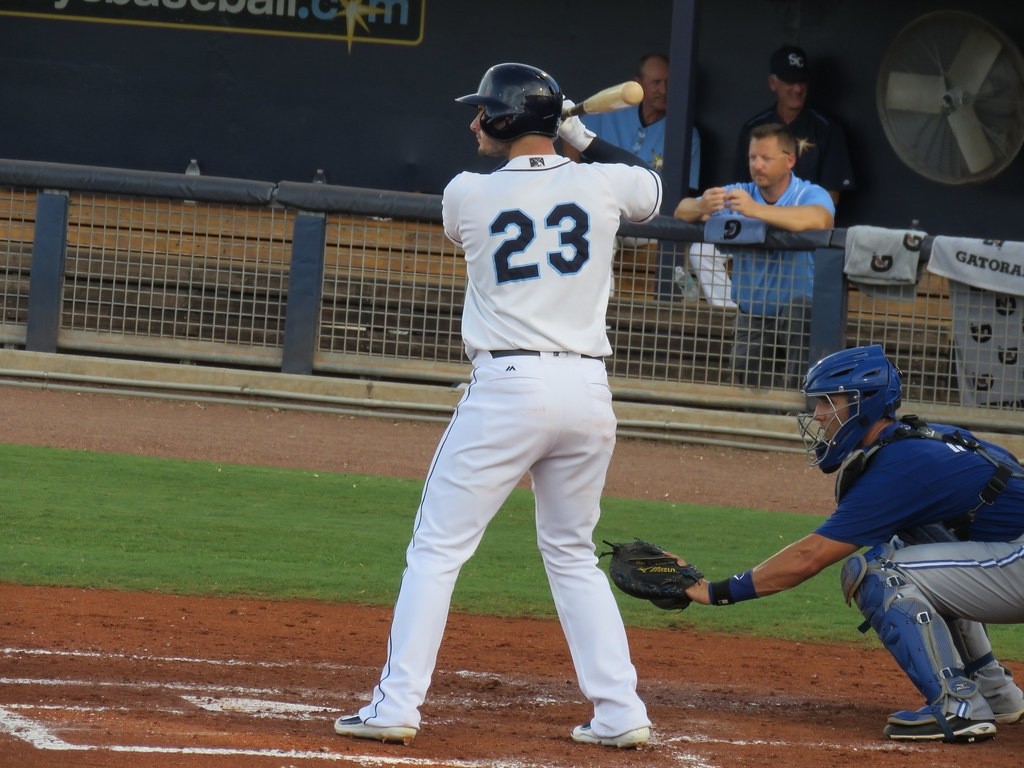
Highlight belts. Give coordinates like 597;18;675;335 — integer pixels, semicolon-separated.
490;348;603;361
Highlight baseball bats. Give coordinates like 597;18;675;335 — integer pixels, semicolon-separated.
563;79;644;121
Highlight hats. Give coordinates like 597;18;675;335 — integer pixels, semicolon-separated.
769;45;810;83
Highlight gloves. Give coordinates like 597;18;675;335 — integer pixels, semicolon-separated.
556;95;597;153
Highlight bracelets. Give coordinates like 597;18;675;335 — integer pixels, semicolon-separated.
708;569;759;606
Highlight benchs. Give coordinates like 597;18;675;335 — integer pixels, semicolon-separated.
0;185;955;396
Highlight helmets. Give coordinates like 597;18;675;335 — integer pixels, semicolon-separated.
797;343;903;475
454;62;563;142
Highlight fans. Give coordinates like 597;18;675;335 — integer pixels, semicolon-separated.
877;10;1024;184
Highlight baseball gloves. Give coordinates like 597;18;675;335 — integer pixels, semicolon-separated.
607;537;703;614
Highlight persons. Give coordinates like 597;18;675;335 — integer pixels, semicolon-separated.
334;62;670;747
563;52;700;220
672;123;834;391
598;344;1024;740
738;44;853;228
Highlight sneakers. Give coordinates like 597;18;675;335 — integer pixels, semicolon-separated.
884;685;1024;740
334;714;418;745
571;719;650;748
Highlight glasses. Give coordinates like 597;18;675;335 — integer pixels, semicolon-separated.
633;126;646;155
